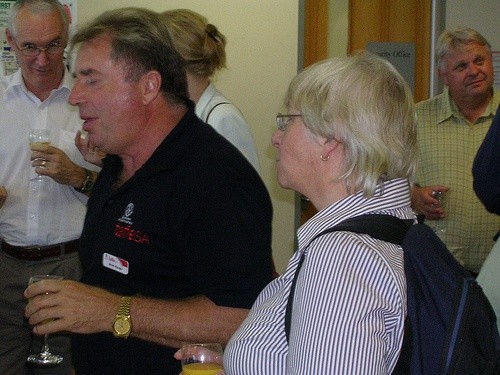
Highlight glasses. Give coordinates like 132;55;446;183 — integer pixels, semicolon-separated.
14;37;66;57
275;113;302;131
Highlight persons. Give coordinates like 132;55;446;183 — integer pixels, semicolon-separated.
408;26;500;281
74;8;260;173
25;8;274;375
0;0;103;375
175;52;417;375
471;102;500;215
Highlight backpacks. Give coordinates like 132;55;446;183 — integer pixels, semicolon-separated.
285;214;500;375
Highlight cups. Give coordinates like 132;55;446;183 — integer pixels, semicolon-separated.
180;341;224;375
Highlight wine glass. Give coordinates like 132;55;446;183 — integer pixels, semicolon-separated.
28;129;50;182
26;275;63;365
430;188;449;234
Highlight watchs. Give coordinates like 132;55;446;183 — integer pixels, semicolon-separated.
112;296;134;339
74;170;94;194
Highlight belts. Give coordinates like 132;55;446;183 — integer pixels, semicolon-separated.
0;238;79;262
